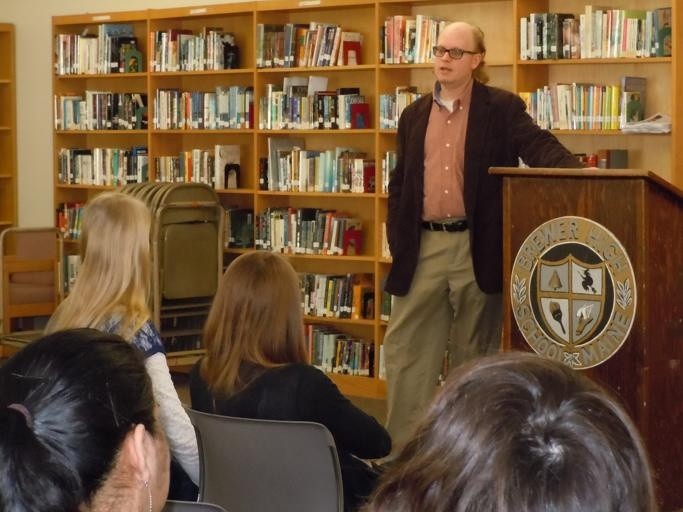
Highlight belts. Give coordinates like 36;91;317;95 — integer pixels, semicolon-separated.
420;219;469;232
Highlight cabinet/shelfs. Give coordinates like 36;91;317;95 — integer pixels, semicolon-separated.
50;0;683;403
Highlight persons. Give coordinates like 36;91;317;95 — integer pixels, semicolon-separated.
43;192;202;503
383;22;598;470
0;327;172;512
184;251;392;512
360;350;660;512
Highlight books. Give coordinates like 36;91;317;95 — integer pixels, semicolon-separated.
379;86;426;130
520;4;673;60
259;136;375;255
154;143;241;190
223;207;254;250
150;27;235;73
152;85;253;130
295;272;377;375
254;23;369;130
518;75;646;129
382;151;395;257
57;201;86;296
54;22;148;130
57;146;149;186
379;15;446;65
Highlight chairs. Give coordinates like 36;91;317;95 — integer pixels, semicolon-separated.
3;227;58;335
180;405;346;511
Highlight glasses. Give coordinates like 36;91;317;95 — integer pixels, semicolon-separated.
433;46;479;60
